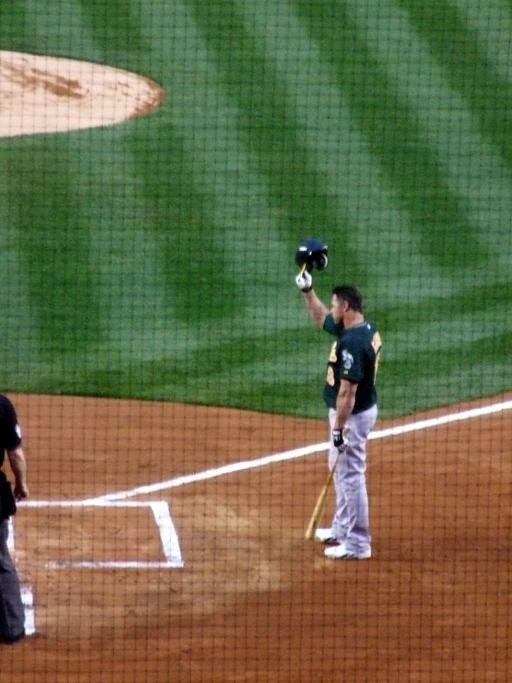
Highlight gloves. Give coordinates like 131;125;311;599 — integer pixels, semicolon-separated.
332;426;351;454
294;270;313;294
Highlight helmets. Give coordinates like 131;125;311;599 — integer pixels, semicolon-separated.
295;238;330;273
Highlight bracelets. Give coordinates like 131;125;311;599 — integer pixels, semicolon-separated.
331;429;344;448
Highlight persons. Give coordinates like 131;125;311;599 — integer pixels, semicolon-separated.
0;393;30;642
295;269;383;561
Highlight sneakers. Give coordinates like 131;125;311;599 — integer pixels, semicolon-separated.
323;542;372;561
314;527;341;544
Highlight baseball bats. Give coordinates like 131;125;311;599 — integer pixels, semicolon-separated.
305;451;341;539
